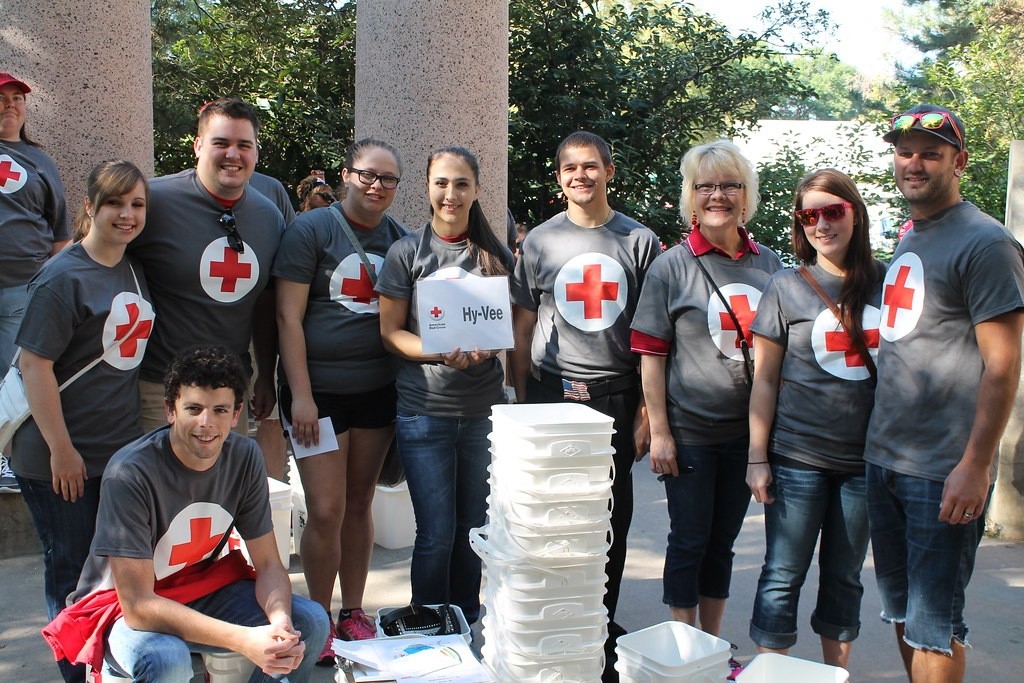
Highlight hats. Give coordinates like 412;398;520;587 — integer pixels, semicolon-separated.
0;72;31;93
882;104;965;151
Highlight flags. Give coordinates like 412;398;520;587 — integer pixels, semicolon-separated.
562;379;590;401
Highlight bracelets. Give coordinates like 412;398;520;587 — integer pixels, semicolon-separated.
747;461;768;464
440;353;443;359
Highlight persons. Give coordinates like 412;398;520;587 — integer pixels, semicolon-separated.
629;141;785;680
296;170;337;212
8;157;156;683
65;348;330;683
373;145;518;625
0;70;69;494
247;172;298;483
60;99;283;433
506;208;528;257
745;169;890;667
513;131;662;622
271;139;409;667
863;104;1024;683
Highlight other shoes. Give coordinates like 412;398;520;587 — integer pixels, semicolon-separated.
728;659;744;683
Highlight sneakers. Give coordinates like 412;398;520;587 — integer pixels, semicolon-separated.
336;606;378;640
0;455;22;494
313;617;339;666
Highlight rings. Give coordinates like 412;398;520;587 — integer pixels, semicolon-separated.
486;351;490;358
965;513;972;516
656;466;661;469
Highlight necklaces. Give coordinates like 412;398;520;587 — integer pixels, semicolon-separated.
605;209;612;223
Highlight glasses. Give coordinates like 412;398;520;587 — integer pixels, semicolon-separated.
794;201;854;226
217;207;244;255
890;114;963;149
312;192;335;204
692;182;744;196
347;167;401;189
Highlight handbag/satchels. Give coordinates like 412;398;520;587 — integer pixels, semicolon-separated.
0;365;35;457
381;602;461;637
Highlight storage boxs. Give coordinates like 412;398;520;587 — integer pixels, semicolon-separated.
329;403;850;683
